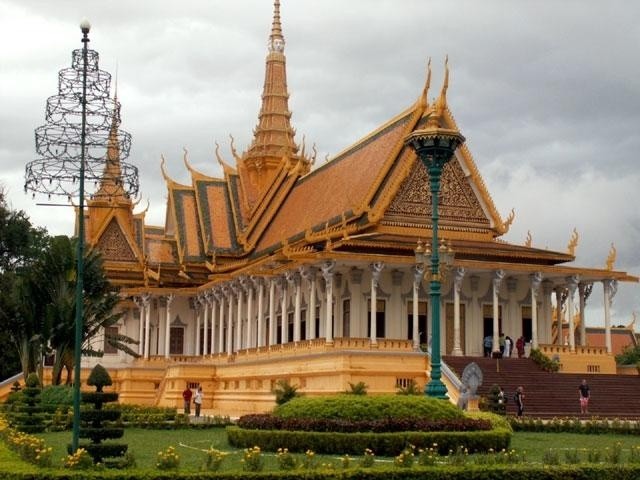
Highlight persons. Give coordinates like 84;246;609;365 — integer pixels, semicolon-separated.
182;384;192;414
498;388;507;411
482;332;526;359
193;386;204;417
575;379;591;414
514;386;525;418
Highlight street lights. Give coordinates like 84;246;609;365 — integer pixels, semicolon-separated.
21;18;141;455
401;128;468;401
